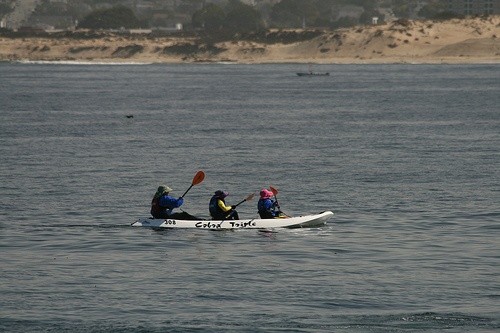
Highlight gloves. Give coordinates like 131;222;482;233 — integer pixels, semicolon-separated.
231;205;236;209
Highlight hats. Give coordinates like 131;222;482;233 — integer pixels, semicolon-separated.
260;189;273;199
213;190;229;199
155;185;173;199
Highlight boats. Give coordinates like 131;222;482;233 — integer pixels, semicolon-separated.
130;210;335;229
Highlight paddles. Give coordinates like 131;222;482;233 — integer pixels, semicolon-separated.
182;171;205;198
235;193;256;207
270;186;278;207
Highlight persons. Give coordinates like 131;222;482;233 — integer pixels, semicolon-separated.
209;189;239;221
258;189;287;218
151;185;203;220
